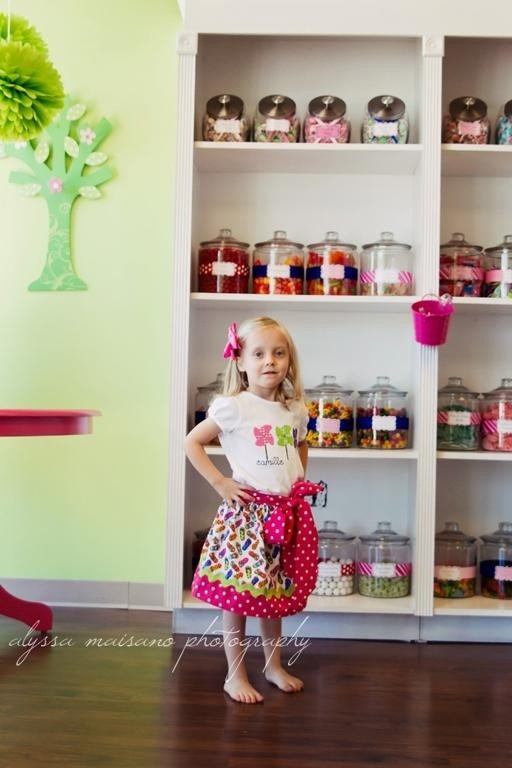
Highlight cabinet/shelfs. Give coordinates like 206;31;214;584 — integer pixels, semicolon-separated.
430;35;512;643
163;32;430;645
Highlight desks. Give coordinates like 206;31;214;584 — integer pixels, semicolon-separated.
0;408;100;639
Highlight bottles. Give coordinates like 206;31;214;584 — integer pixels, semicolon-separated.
252;94;300;143
355;521;411;598
437;377;481;450
252;230;305;294
194;373;223;445
439;232;485;297
192;528;209;578
479;521;512;600
484;234;512;299
494;99;512;145
434;522;478;598
481;378;512;452
306;231;358;295
302;95;351;143
442;96;491;144
360;95;409;143
197;228;250;293
356;376;409;449
312;521;355;596
359;232;415;296
304;375;354;448
202;94;251;142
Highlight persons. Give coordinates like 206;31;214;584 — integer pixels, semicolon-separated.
186;316;320;704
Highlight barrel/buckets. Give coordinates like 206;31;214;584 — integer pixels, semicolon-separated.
408;292;455;348
